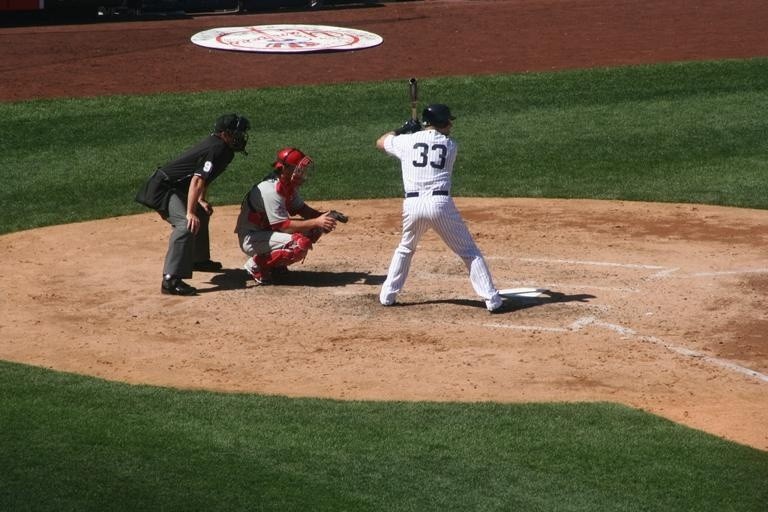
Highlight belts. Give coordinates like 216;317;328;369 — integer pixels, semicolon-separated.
402;192;449;198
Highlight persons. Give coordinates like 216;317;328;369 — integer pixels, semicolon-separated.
233;146;337;286
133;111;251;297
375;104;513;314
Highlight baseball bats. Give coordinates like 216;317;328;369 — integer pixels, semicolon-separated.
410;78;417;119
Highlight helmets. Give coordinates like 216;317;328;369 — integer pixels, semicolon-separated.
420;102;459;127
214;112;250;157
271;147;313;186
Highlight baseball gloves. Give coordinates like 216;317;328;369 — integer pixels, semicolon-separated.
319;211;348;233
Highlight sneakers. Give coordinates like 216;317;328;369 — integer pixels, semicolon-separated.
243;258;271;285
485;302;507;313
160;275;198;296
191;260;223;271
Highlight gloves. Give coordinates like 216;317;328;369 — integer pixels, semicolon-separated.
394;118;420;134
